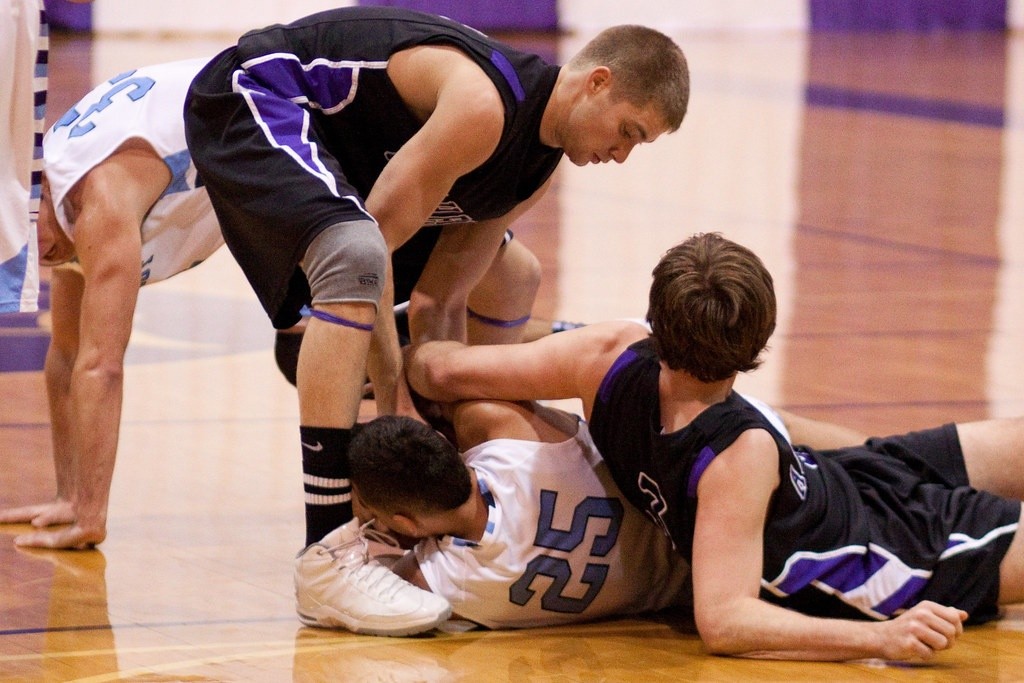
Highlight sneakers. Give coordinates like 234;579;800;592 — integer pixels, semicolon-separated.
293;516;454;638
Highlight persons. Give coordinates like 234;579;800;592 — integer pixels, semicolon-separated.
185;3;692;635
2;56;587;549
348;399;871;638
394;232;1024;665
